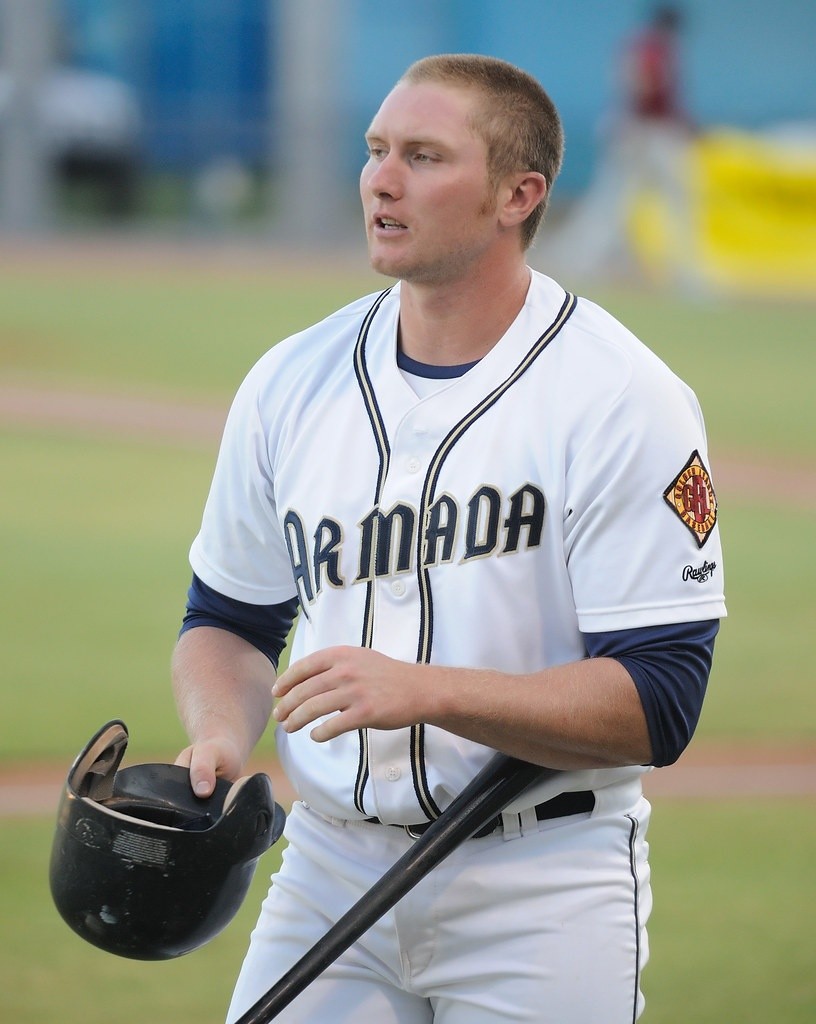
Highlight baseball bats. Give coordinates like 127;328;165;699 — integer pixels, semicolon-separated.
233;749;556;1024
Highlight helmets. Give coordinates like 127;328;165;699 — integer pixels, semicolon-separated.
48;718;286;961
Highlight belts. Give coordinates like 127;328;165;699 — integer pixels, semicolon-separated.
363;790;596;838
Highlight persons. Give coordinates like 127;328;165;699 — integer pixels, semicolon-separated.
171;51;730;1024
613;0;703;278
